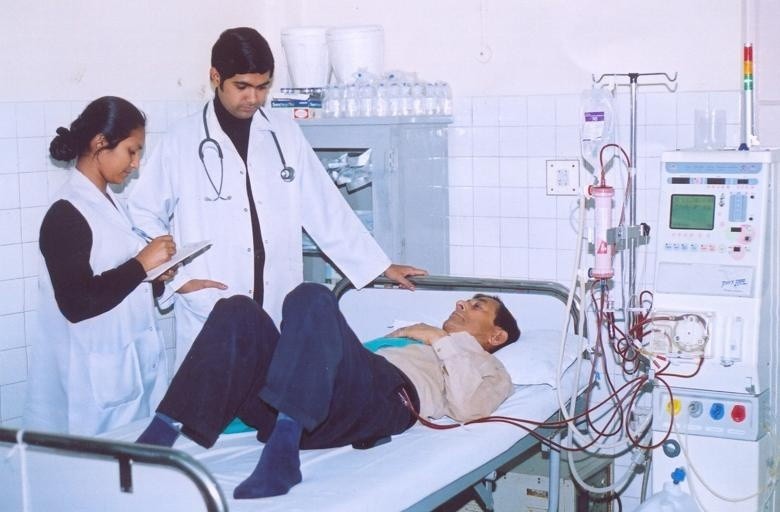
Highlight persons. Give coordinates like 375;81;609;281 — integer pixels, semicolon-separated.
125;27;429;389
134;281;519;498
38;96;178;437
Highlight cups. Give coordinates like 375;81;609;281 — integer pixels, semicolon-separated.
695;107;726;152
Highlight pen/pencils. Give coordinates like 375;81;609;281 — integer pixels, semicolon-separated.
132;227;151;242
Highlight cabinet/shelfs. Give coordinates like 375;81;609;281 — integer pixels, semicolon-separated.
291;116;453;288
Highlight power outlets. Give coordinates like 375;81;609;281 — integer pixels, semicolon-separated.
546;159;579;196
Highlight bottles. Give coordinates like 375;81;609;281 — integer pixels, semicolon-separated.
323;82;453;117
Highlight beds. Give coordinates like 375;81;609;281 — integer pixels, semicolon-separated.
2;276;594;512
